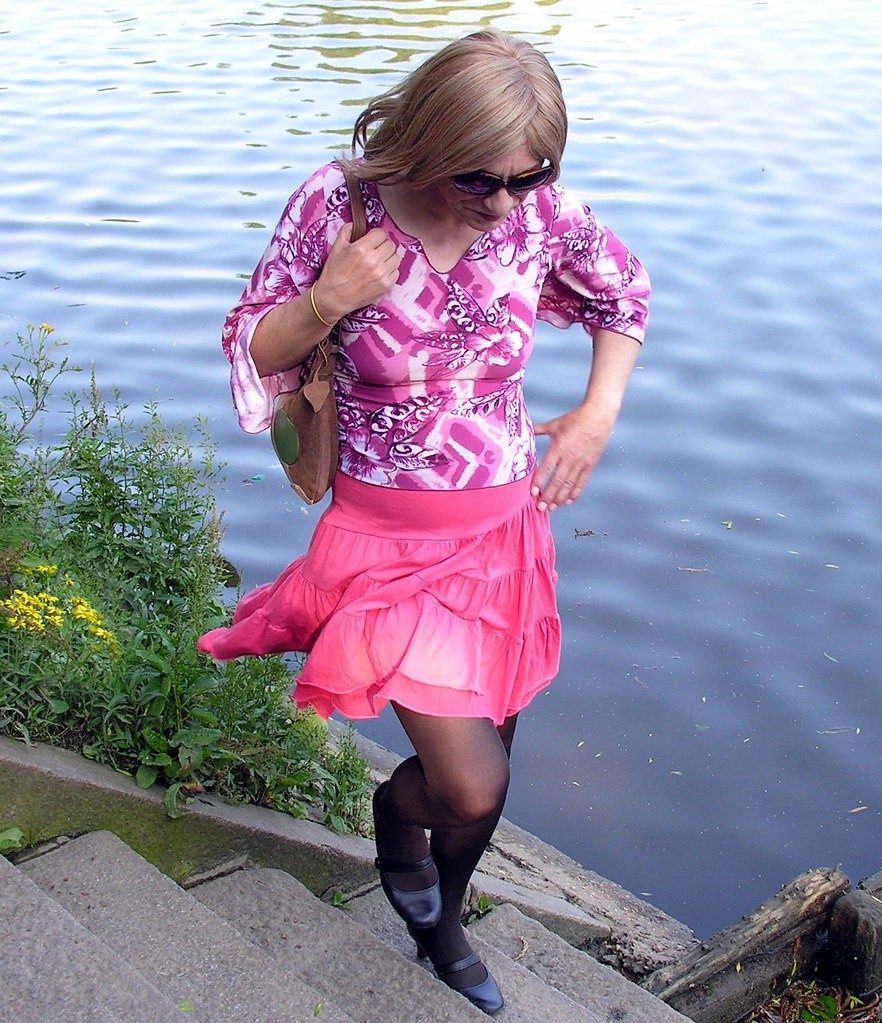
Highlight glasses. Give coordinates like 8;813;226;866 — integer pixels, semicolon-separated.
449;161;555;196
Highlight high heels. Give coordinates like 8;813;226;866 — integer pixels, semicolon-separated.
408;923;504;1015
372;781;441;930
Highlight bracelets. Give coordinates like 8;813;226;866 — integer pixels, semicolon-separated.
311;281;337;326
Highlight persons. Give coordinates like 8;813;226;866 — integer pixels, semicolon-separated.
196;29;652;1013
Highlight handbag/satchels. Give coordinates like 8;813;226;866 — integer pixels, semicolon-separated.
268;160;365;505
